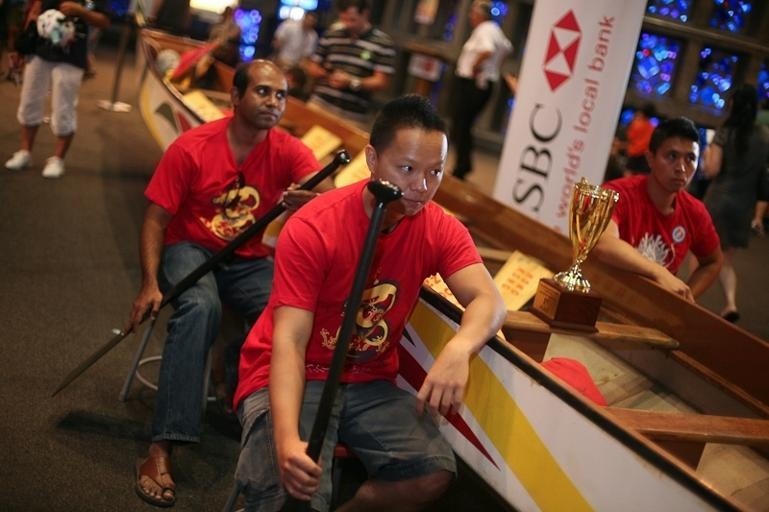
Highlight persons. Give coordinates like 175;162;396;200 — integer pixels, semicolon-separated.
120;56;335;503
609;58;768;251
232;93;510;512
82;1;162;78
5;0;111;181
593;115;726;306
304;2;403;130
210;2;317;89
450;1;515;183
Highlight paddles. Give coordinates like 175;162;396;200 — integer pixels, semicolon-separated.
51;150;352;395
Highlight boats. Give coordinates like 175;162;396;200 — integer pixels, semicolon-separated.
133;25;769;512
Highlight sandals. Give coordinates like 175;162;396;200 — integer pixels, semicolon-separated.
210;368;236;420
136;446;177;507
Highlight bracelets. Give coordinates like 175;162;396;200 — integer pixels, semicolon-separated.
350;80;361;91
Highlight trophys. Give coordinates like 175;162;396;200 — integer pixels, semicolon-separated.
554;172;620;299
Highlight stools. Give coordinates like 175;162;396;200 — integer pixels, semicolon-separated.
115;281;248;427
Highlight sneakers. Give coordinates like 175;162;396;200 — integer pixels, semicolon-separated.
42;156;66;179
5;150;32;170
